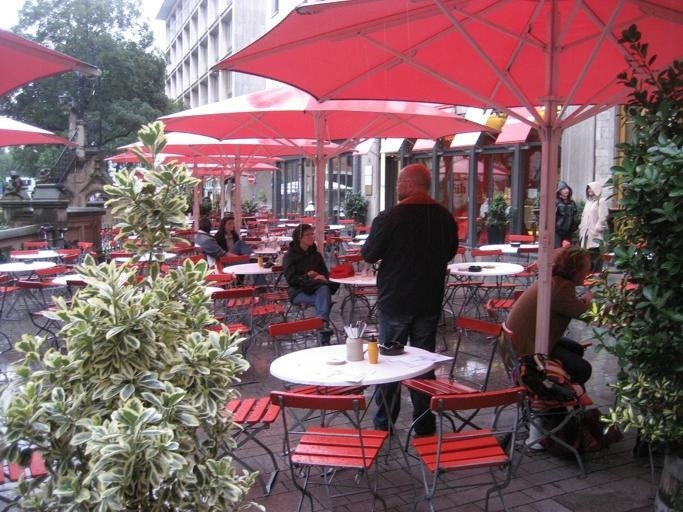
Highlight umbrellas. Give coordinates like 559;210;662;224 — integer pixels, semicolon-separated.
0;27;102;95
156;85;502;260
1;114;80;149
104;154;286;231
118;132;359;236
172;163;279;220
210;0;683;452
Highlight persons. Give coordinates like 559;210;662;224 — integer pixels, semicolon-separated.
504;245;599;384
579;180;601;243
215;216;240;252
359;162;459;441
554;181;581;250
193;218;239;258
282;224;334;348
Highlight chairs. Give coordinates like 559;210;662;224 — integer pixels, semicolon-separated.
391;316;502;473
449;246;483;306
471;249;517;300
513;247;538;283
206;274;260;309
498;322;593;482
270;390;391;512
508;234;535;245
213;396;280;498
251;265;285;358
209;288;255;359
268;317;370;430
483;298;515;321
411;387;529;512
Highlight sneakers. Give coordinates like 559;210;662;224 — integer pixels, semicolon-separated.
320;322;333;336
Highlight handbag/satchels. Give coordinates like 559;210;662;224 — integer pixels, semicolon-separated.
328;262;355;279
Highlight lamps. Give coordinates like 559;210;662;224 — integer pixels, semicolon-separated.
304;201;315;212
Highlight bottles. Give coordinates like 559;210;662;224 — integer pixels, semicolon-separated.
368;335;378;363
259;256;262;267
